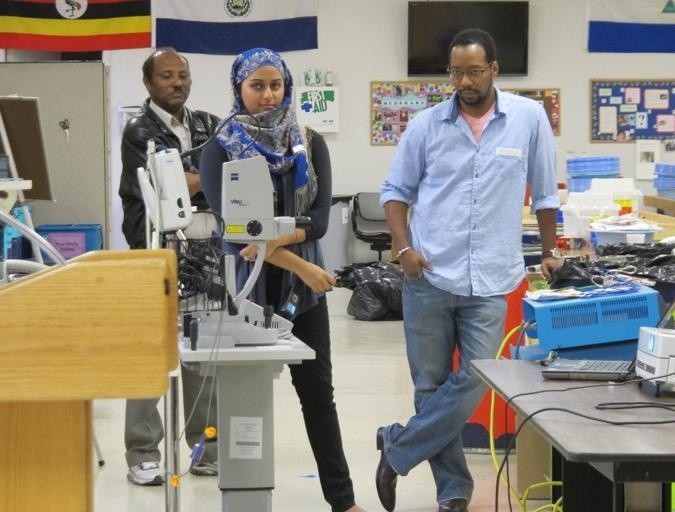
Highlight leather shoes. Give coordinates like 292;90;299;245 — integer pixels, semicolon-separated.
439;497;469;512
376;425;398;512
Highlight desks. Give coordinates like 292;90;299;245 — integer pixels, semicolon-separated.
468;357;675;512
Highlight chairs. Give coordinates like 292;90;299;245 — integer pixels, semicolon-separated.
351;191;392;261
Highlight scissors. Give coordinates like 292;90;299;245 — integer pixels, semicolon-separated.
59;119;73;142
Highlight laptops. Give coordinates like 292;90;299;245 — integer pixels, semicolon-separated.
540;299;675;383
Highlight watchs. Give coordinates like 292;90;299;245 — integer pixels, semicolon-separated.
540;246;563;260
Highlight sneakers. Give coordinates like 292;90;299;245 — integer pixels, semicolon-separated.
129;460;166;487
190;462;220;477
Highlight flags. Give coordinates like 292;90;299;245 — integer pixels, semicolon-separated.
0;1;152;53
584;1;675;55
154;1;319;56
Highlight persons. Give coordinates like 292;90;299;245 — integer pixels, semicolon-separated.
116;46;221;486
374;27;567;512
199;46;370;510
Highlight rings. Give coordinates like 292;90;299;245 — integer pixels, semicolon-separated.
243;254;249;261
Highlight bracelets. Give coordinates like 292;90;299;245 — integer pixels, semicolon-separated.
293;227;300;247
395;246;412;259
